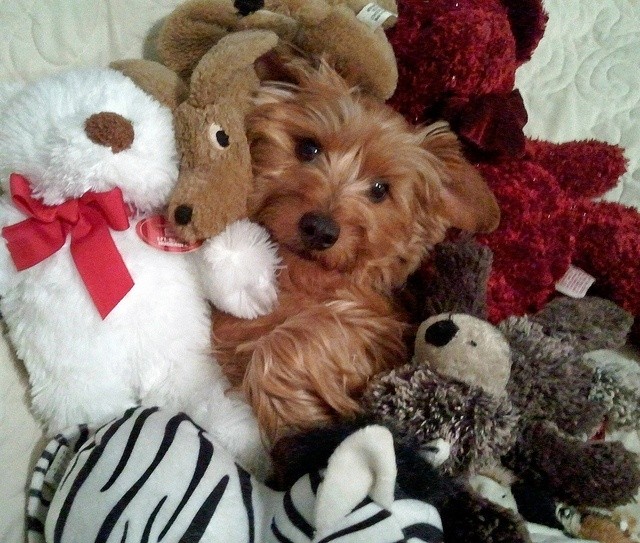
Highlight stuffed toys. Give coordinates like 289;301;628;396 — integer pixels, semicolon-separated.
387;0;639;324
110;0;399;242
26;407;445;542
0;65;278;480
358;225;640;543
